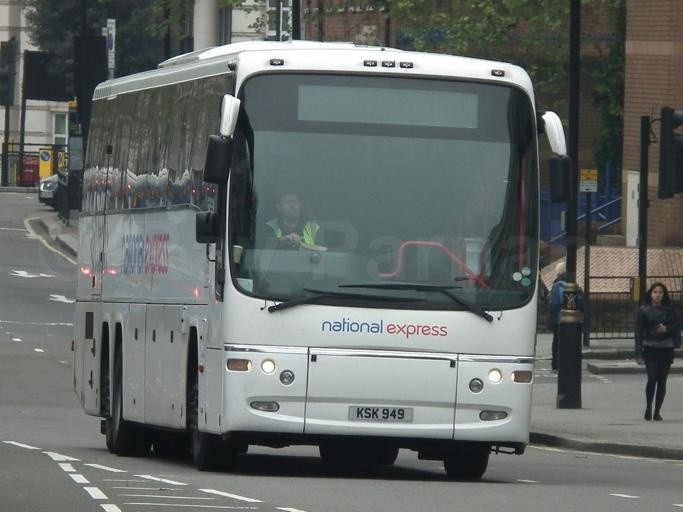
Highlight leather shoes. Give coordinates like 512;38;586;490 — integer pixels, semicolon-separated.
645;409;663;420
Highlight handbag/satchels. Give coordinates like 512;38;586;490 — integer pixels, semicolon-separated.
673;331;681;348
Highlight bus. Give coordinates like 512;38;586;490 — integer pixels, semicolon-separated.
70;34;581;484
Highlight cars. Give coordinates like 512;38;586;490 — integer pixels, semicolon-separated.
37;173;58;210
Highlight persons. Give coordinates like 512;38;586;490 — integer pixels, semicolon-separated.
261;190;327;251
634;283;680;421
546;262;587;372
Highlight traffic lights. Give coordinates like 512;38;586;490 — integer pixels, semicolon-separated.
656;105;683;201
